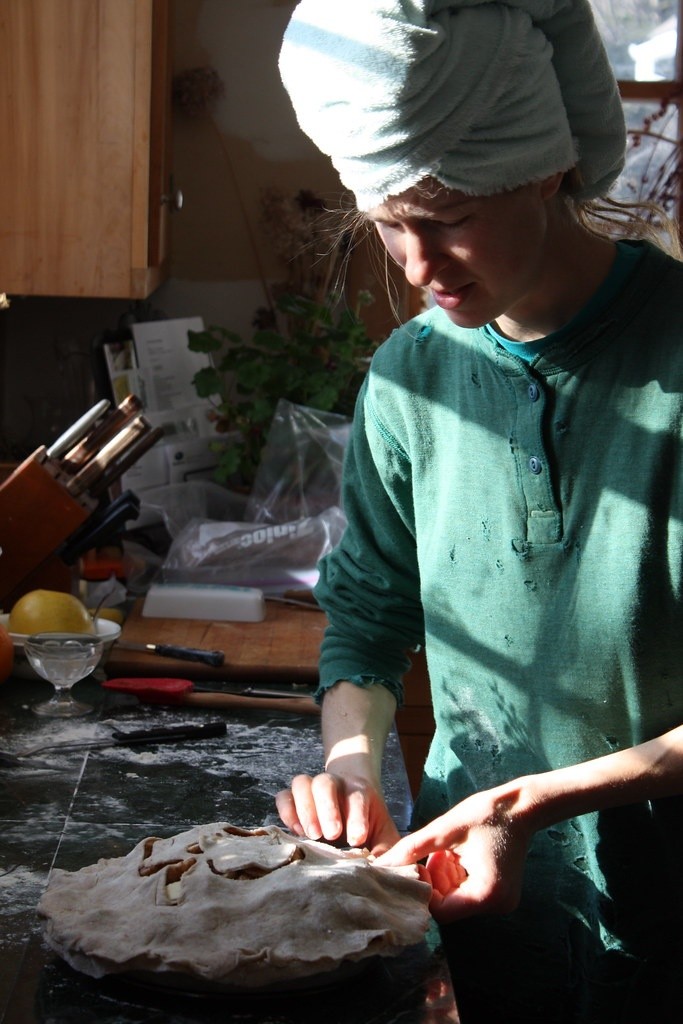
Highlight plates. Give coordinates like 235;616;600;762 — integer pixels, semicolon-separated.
0;613;121;648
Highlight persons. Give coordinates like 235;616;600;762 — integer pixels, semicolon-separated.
275;0;683;1024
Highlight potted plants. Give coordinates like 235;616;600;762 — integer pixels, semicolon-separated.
184;290;388;523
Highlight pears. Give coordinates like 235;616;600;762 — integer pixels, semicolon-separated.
7;588;96;636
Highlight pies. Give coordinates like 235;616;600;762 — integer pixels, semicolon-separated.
36;821;435;983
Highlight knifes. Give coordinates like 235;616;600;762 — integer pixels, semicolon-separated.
112;642;227;666
36;393;171;568
18;724;230;759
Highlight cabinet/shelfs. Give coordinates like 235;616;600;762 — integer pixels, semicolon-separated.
0;0;184;299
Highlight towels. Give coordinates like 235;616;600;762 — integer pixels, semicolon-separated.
277;0;628;213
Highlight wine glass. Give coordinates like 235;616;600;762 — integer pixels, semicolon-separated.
25;629;104;718
